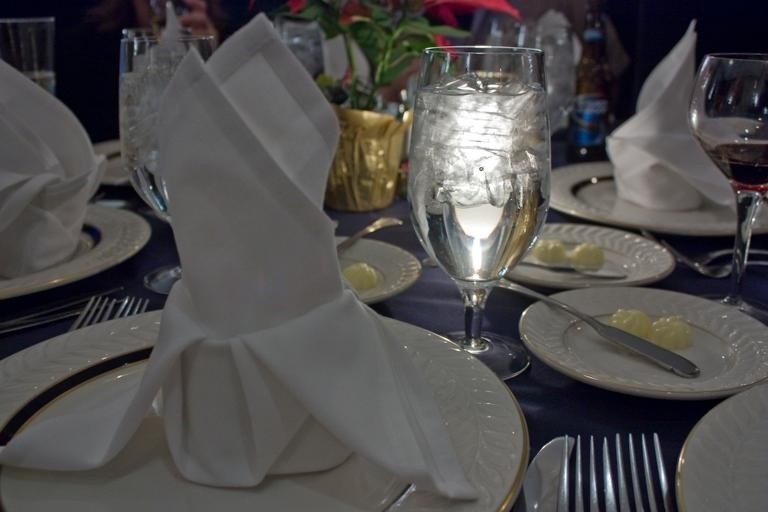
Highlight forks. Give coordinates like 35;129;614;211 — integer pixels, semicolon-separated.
71;296;149;327
558;433;674;512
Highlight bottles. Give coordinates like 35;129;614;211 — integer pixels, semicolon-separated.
565;29;611;163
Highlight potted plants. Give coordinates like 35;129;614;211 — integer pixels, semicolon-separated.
283;0;477;211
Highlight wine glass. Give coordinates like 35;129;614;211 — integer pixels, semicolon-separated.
121;33;218;296
407;45;551;380
687;53;768;322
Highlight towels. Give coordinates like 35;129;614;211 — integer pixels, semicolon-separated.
602;19;742;211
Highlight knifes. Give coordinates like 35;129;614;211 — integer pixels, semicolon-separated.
3;302;82;330
498;275;701;380
527;434;573;512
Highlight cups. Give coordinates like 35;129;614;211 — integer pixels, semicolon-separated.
0;17;56;95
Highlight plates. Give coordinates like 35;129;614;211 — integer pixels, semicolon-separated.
507;223;676;288
677;381;768;511
331;232;420;304
1;201;150;296
88;141;129;189
543;163;767;238
1;311;528;511
520;285;768;399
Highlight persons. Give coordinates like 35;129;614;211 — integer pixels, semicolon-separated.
91;0;230;52
468;0;631;123
